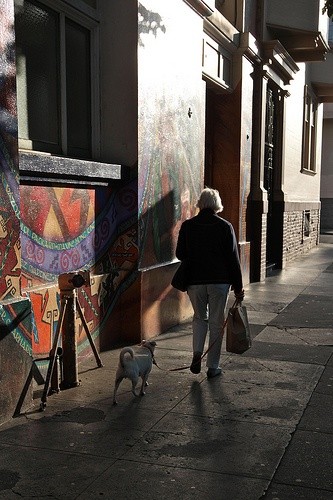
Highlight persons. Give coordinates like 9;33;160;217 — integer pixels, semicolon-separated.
170;188;245;377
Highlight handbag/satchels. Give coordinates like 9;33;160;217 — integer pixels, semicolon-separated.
170;262;189;293
224;296;253;355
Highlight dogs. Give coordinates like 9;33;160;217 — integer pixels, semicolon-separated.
113;340;156;407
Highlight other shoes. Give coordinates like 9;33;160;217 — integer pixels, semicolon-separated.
189;352;203;374
206;364;222;379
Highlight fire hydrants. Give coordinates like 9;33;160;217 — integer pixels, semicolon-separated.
40;270;105;411
